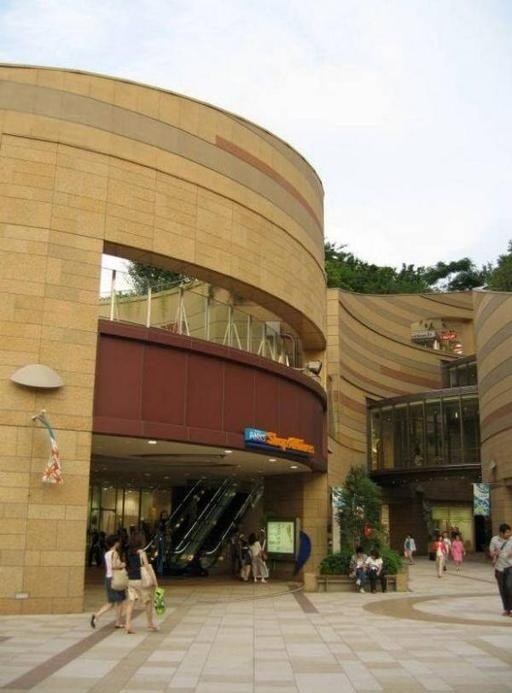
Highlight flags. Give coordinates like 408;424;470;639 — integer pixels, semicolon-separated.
34;409;65;487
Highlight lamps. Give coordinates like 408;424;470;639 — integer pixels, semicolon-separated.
12;365;63;390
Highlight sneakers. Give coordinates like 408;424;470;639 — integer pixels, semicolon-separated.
503;610;512;617
356;579;364;592
90;614;96;628
116;623;135;634
147;624;159;631
372;589;386;593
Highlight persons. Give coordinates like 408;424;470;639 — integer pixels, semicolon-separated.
248;533;270;584
88;527;146;568
349;546;368;594
125;533;161;635
361;549;387;595
90;535;127;629
403;533;417;565
432;532;466;578
239;534;252;581
489;524;512;618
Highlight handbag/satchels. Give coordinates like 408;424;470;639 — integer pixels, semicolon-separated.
140;564;157;587
111;568;128;591
492;551;497;565
260;551;267;561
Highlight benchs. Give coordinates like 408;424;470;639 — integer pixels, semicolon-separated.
302;573;404;593
